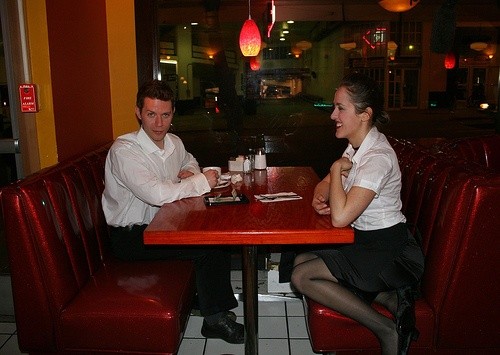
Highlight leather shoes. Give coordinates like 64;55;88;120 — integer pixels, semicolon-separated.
200;317;245;344
191;295;236;321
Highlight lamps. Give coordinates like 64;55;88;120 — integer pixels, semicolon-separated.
238;10;261;56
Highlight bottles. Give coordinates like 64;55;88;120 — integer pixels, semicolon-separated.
255;147;266;170
248;149;255;171
243;156;252;174
243;171;268;189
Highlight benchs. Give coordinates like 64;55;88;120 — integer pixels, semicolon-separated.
0;141;196;355
304;132;500;354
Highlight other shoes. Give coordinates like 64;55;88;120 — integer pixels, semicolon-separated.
396;285;421;338
397;327;420;355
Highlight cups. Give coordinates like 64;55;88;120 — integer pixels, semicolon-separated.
203;167;222;176
228;160;243;171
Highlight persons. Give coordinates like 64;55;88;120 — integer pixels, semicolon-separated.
98;80;246;345
291;73;424;355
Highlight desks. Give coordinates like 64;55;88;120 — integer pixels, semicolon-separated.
144;166;355;354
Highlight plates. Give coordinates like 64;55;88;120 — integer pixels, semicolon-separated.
214;175;231;188
